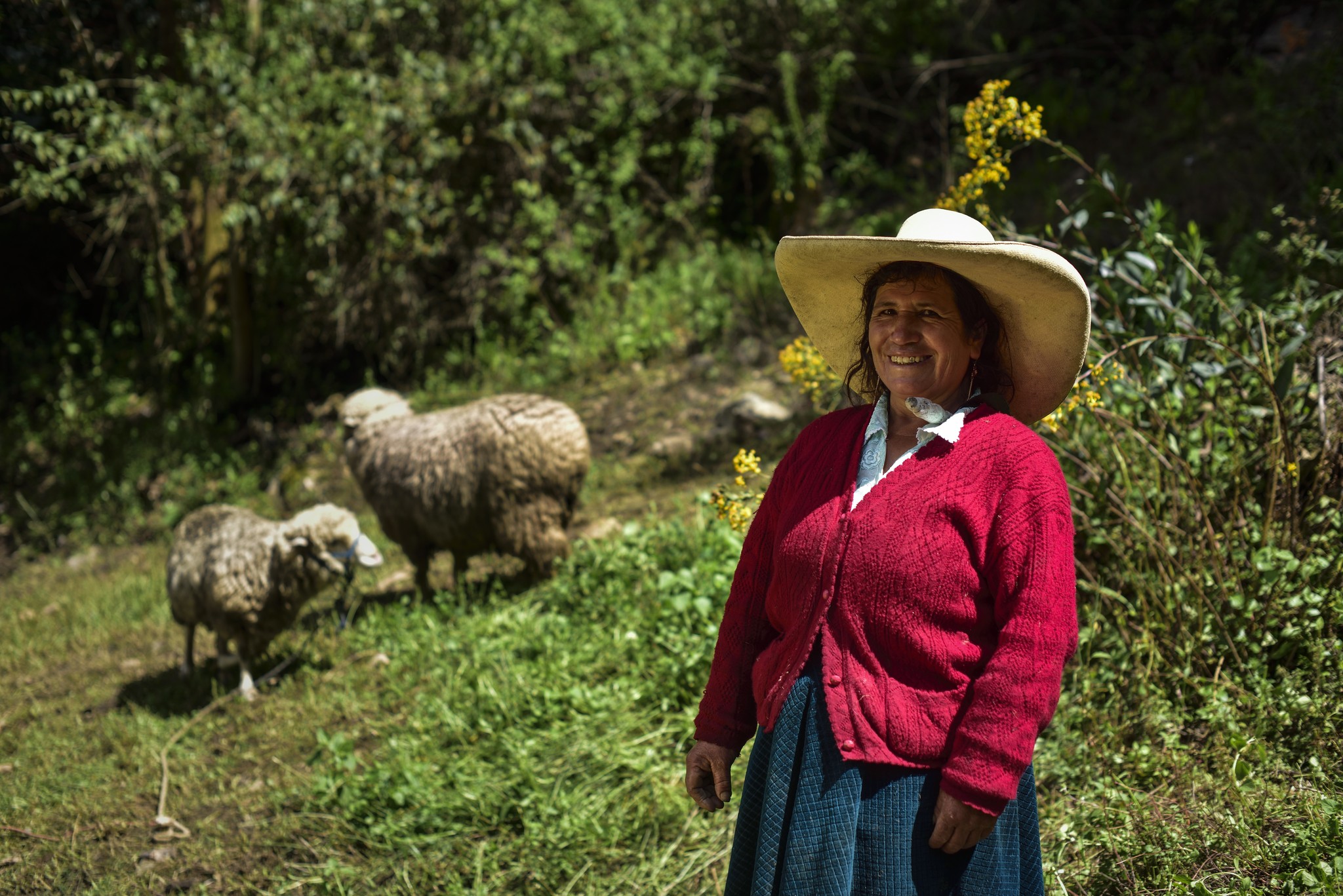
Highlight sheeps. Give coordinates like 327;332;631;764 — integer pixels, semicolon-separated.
162;500;386;708
333;382;594;607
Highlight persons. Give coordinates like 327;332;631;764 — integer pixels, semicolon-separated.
684;207;1091;896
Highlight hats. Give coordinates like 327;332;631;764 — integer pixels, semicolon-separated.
774;209;1091;426
